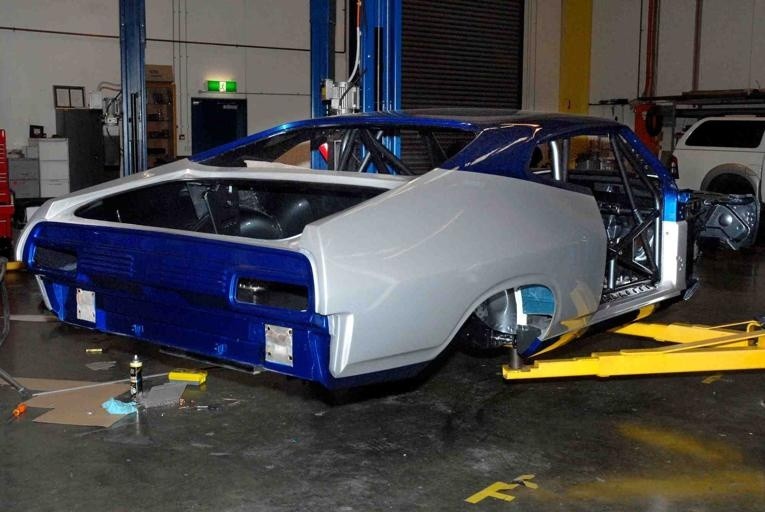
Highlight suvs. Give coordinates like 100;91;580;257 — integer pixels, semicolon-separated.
669;115;765;209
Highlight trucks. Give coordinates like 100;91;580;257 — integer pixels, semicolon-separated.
0;128;14;243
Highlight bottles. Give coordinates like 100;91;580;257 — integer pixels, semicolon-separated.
129;352;143;398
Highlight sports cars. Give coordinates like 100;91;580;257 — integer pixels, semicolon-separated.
17;106;759;391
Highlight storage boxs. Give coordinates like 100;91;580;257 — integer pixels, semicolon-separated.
7;136;71;200
